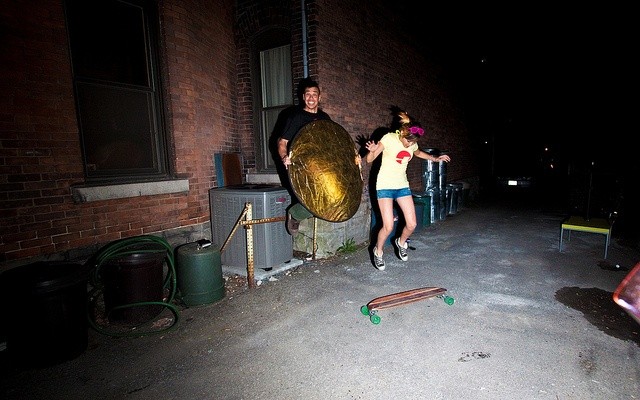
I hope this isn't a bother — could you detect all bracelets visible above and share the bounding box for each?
[281,154,289,161]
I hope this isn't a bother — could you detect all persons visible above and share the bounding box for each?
[365,113,451,271]
[277,82,330,235]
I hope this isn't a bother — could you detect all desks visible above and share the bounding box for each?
[559,215,612,259]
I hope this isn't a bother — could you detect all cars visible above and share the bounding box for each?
[496,151,555,193]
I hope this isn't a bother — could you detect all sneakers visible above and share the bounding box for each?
[288,211,300,237]
[373,246,385,271]
[395,237,408,261]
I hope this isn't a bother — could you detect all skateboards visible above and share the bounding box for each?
[360,286,455,324]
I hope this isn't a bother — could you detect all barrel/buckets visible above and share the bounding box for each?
[411,202,424,233]
[423,192,440,223]
[420,147,439,192]
[0,259,91,371]
[98,240,166,326]
[176,241,224,307]
[439,161,448,190]
[370,207,401,248]
[414,193,431,227]
